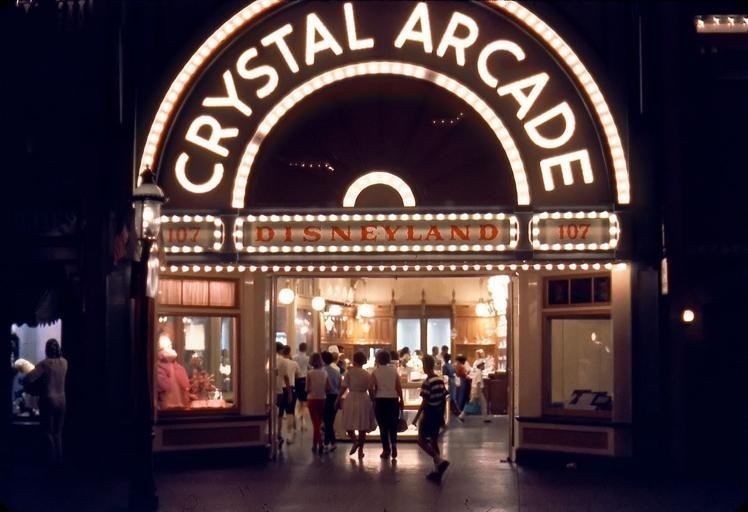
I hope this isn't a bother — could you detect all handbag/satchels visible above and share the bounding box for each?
[464,398,481,415]
[398,409,407,432]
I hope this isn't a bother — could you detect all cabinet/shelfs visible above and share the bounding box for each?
[347,379,425,442]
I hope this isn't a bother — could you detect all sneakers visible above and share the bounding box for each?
[426,461,449,480]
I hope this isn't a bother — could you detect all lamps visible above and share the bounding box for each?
[475,275,508,345]
[279,275,377,331]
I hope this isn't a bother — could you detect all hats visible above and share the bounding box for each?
[328,345,339,353]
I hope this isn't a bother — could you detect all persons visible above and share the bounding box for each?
[266,341,491,483]
[18,339,68,457]
